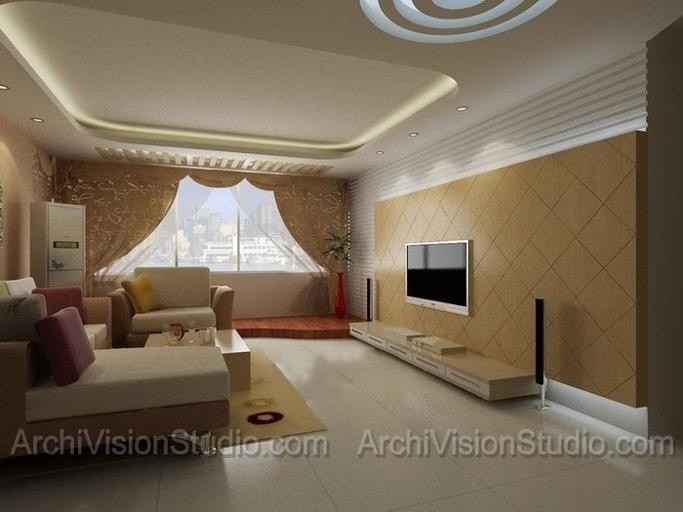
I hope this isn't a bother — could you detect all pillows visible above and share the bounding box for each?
[121,273,164,313]
[32,286,87,325]
[1,292,47,343]
[34,306,96,386]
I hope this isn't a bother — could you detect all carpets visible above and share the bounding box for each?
[0,345,328,479]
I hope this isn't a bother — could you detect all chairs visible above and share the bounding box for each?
[108,267,235,346]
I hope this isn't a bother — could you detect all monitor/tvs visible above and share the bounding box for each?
[404,239,473,317]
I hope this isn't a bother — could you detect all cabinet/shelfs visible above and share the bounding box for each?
[349,321,541,401]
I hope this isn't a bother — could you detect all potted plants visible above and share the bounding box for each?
[322,231,354,319]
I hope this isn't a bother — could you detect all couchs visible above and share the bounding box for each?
[1,277,113,349]
[0,340,230,458]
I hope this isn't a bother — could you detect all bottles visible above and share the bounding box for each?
[184,327,215,346]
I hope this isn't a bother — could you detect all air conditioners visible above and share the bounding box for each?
[30,201,86,298]
